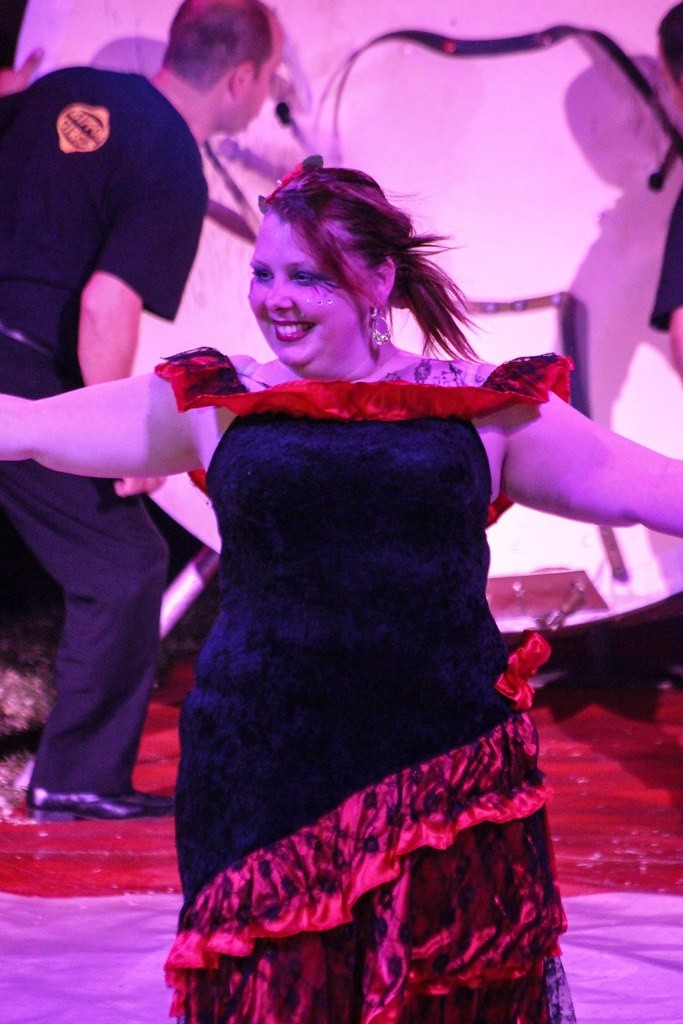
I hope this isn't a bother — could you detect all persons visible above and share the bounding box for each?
[0,151,683,1024]
[0,0,283,818]
[648,1,683,387]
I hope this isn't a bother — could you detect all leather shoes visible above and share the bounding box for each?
[28,786,175,823]
